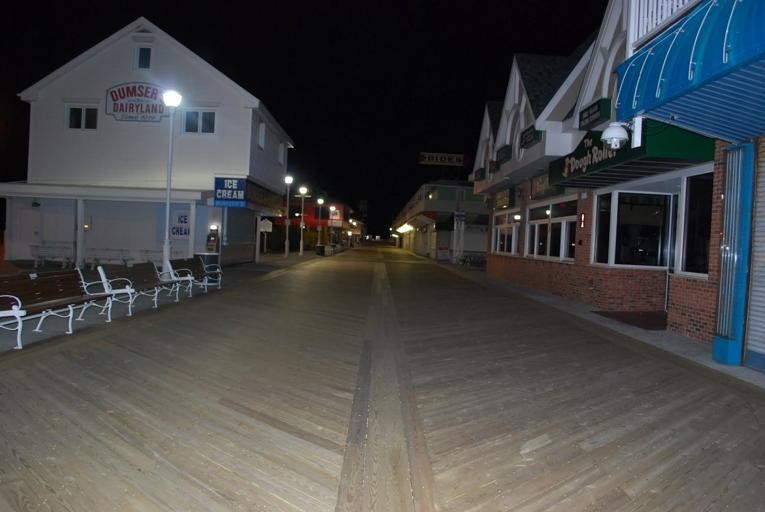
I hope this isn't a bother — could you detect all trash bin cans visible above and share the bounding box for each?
[193,253,219,287]
[315,245,326,256]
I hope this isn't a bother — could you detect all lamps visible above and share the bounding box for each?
[601,121,635,151]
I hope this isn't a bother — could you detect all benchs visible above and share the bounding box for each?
[28,244,74,269]
[0,267,114,349]
[97,261,182,316]
[140,249,185,264]
[85,247,134,270]
[167,257,224,299]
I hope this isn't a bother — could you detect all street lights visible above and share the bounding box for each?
[297,185,307,255]
[159,89,183,284]
[329,206,335,245]
[314,198,325,247]
[283,177,294,258]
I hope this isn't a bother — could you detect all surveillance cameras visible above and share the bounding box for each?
[600,126,629,150]
